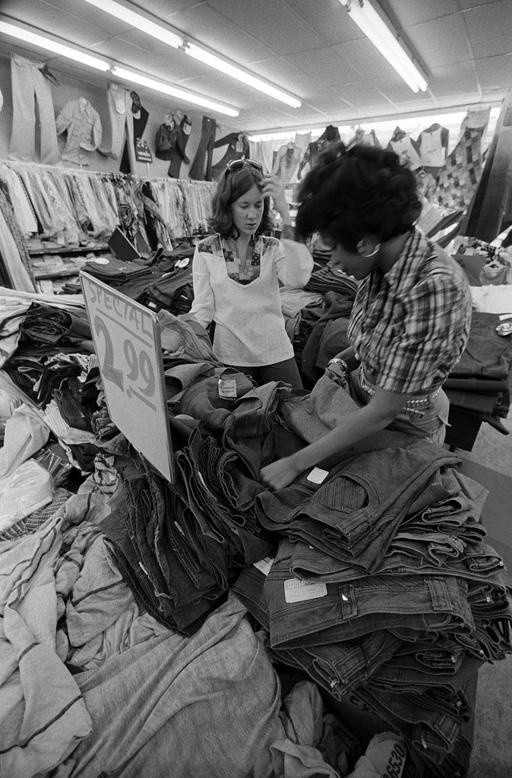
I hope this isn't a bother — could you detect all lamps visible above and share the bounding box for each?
[0,15,110,71]
[86,1,185,55]
[347,0,430,94]
[113,61,241,119]
[185,31,304,110]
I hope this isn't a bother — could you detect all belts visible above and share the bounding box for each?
[358,363,442,420]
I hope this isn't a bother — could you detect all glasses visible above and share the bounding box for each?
[224,158,262,179]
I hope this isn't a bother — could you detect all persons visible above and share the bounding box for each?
[260,143,473,491]
[187,159,313,395]
